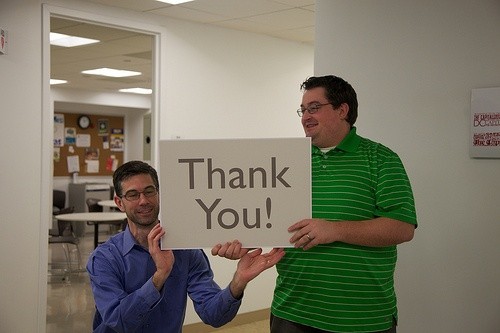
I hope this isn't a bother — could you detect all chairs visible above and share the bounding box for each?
[86,198,121,244]
[49,190,81,282]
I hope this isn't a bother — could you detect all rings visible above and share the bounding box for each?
[306,234,311,240]
[267,260,270,264]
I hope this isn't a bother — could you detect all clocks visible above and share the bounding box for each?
[77,115,91,129]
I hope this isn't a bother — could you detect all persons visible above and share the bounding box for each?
[211,75,419,333]
[85,161,286,333]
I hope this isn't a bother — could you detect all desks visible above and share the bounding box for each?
[55,212,128,250]
[98,200,119,208]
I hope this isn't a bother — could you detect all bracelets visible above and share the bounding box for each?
[152,276,156,284]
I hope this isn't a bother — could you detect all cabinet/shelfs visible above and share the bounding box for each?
[69,183,115,235]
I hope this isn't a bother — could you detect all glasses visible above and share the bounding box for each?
[297,103,332,117]
[120,187,157,200]
